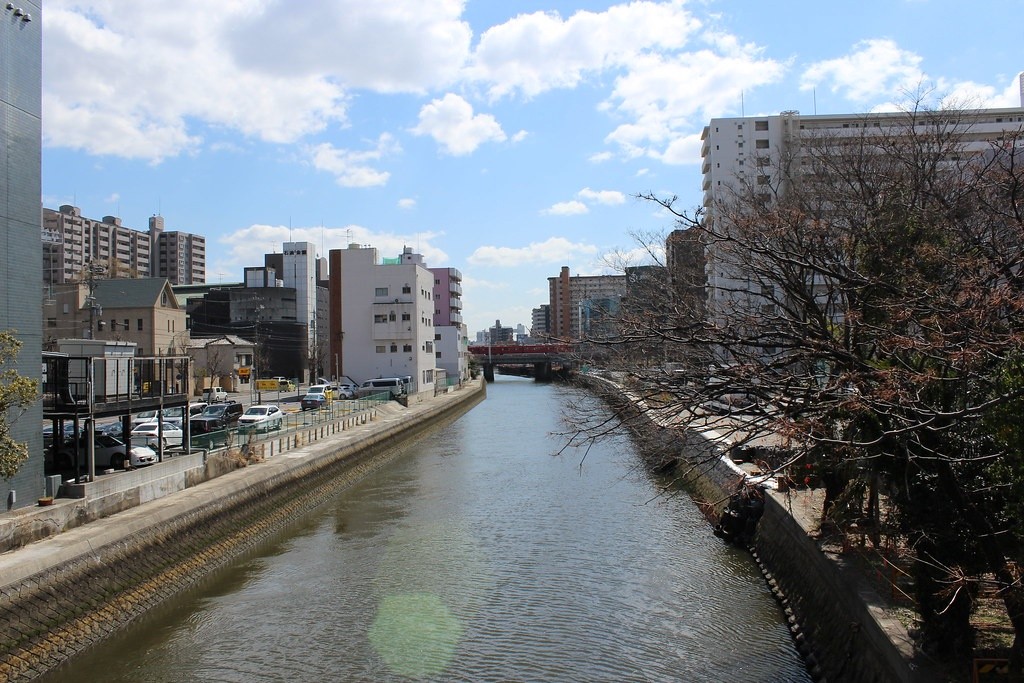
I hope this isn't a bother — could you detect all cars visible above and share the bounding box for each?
[280,380,296,393]
[51,435,156,466]
[307,377,357,400]
[95,421,137,436]
[131,421,183,451]
[300,394,328,411]
[43,423,84,448]
[237,404,283,433]
[133,409,166,427]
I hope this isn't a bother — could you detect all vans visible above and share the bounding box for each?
[358,377,402,397]
[202,387,228,401]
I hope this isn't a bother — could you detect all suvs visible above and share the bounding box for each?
[191,403,243,435]
[164,402,208,429]
